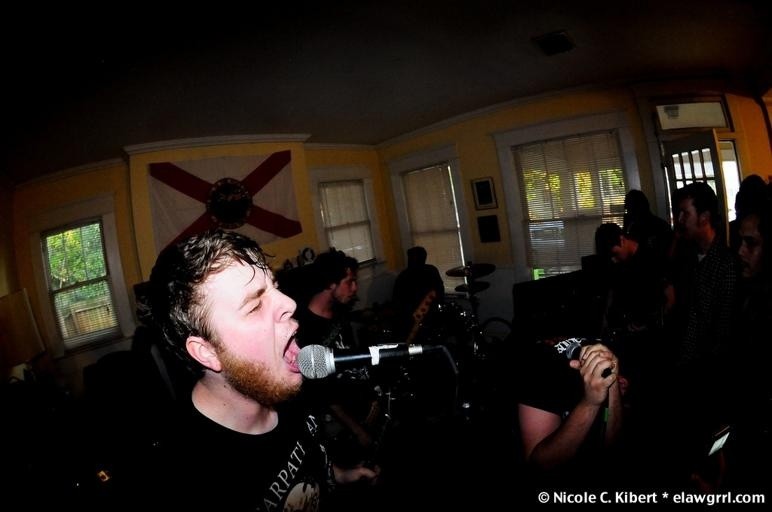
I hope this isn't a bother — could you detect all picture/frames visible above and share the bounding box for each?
[469,176,499,212]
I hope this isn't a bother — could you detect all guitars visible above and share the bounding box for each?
[332,289,443,471]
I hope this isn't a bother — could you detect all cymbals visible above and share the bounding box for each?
[456,281,490,293]
[445,262,496,277]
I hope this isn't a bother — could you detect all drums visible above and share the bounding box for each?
[436,302,466,338]
[422,303,452,343]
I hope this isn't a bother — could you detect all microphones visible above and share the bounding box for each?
[295,343,424,379]
[565,343,613,381]
